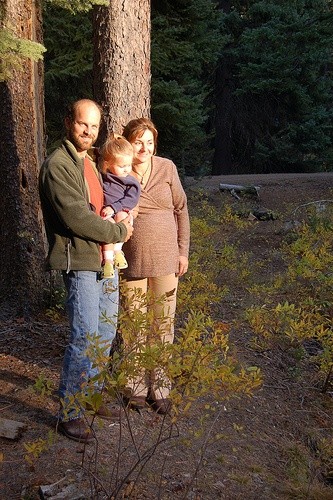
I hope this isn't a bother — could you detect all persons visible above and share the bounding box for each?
[38,98,126,441]
[118,117,190,416]
[94,130,142,281]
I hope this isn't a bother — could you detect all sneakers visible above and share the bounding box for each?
[155,398,173,413]
[86,400,123,420]
[127,395,146,408]
[59,417,94,444]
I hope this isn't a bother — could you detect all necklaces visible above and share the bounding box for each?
[131,159,160,185]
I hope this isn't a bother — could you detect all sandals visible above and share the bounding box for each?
[100,250,128,279]
